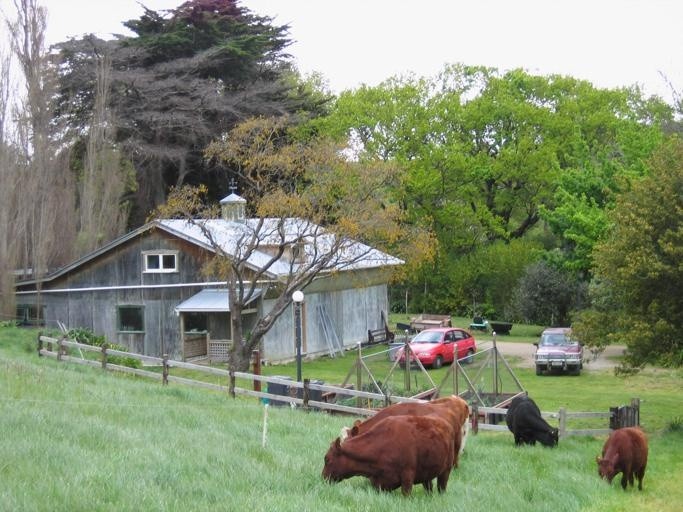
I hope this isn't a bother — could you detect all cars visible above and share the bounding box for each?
[394,326,476,369]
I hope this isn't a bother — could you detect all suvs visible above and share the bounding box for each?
[531,326,585,378]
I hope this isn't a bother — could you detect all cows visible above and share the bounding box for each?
[338,393,471,470]
[320,414,456,500]
[596,426,648,492]
[505,393,559,449]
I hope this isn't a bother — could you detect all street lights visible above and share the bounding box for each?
[291,290,305,400]
